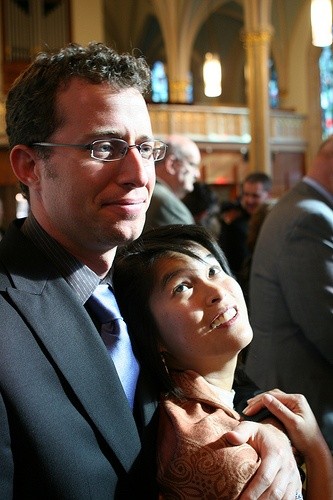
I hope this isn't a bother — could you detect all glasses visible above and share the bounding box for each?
[31,138,168,162]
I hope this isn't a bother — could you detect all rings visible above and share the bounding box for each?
[295,492,303,500]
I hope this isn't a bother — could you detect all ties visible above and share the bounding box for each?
[90,285,140,417]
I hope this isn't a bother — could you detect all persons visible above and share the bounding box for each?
[145,130,333,451]
[0,40,303,500]
[113,224,333,500]
